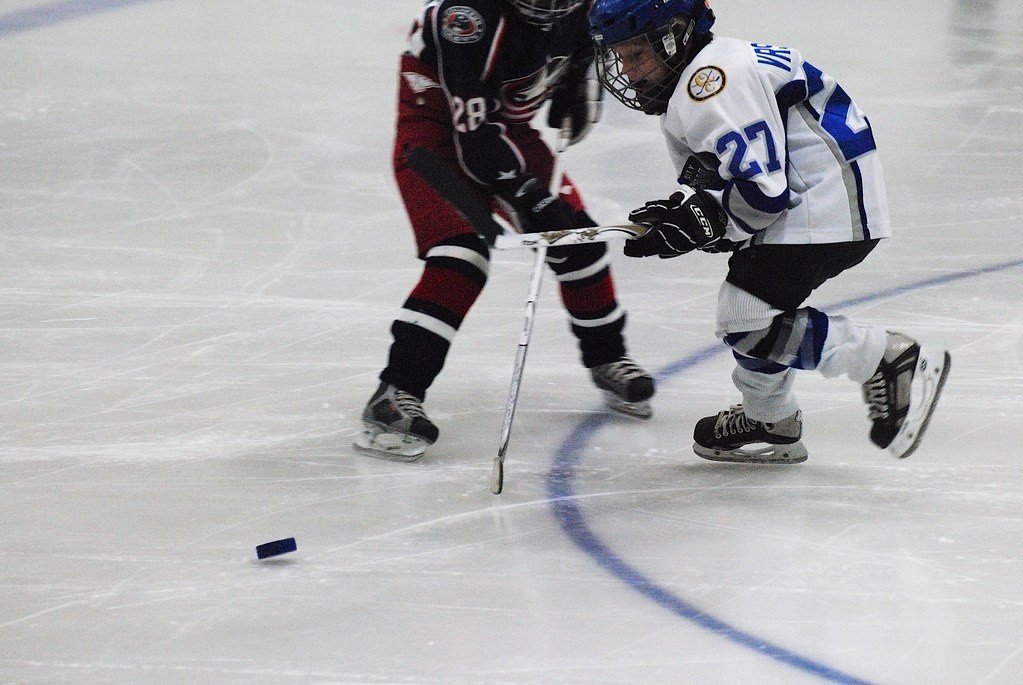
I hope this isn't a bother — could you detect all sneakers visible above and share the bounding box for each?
[353,378,438,463]
[594,357,655,419]
[862,331,950,457]
[692,404,809,465]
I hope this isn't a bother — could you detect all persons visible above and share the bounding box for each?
[587,0,952,464]
[351,0,654,460]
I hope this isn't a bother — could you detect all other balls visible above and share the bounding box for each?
[255,537,298,561]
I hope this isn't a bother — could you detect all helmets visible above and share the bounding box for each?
[587,0,715,116]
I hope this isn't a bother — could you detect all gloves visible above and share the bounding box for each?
[623,191,727,260]
[497,180,585,272]
[548,66,588,145]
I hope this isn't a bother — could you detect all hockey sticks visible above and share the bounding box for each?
[409,144,659,252]
[489,114,572,496]
